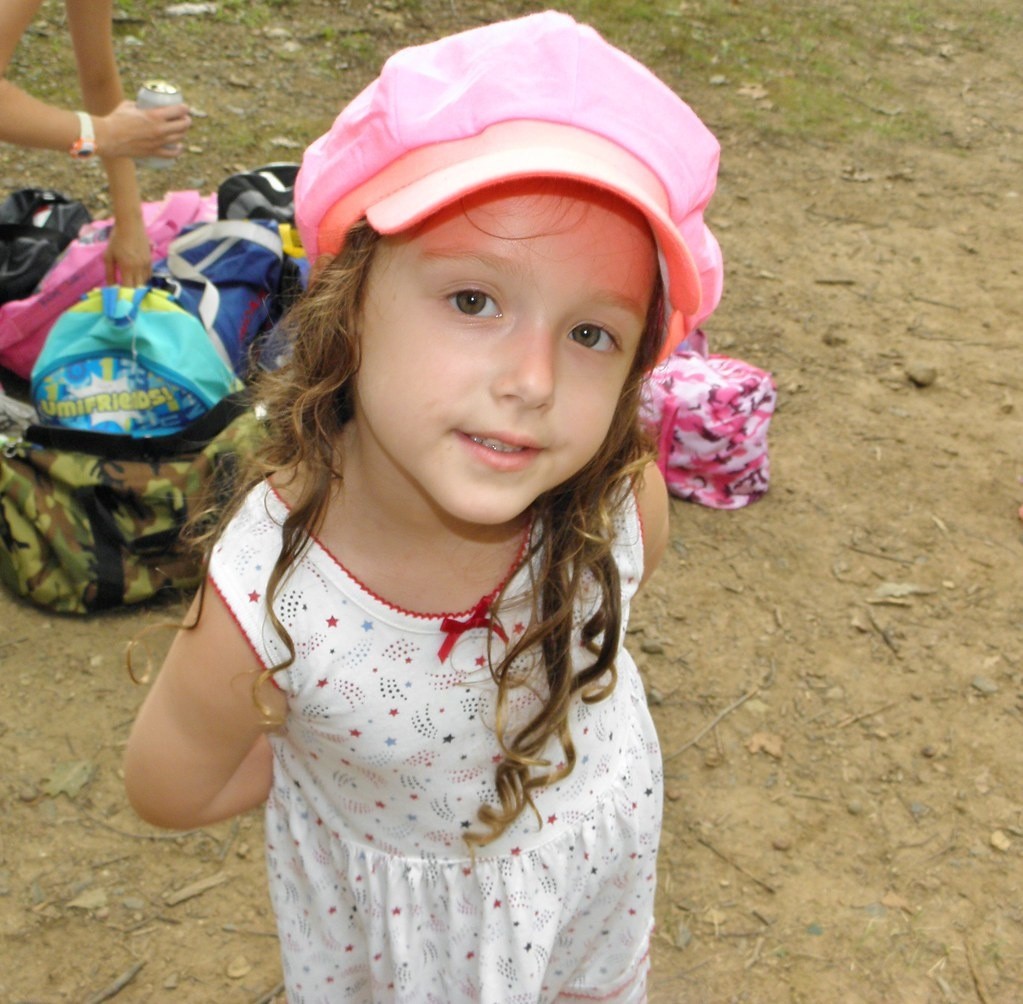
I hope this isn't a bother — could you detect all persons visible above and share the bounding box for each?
[0,0,191,290]
[124,8,721,1004]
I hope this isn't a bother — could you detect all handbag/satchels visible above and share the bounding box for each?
[637,331,776,511]
[0,163,303,614]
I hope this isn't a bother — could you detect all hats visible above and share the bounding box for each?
[294,9,723,375]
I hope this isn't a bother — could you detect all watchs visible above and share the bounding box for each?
[70,112,97,158]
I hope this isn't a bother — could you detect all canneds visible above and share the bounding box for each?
[133,79,184,169]
